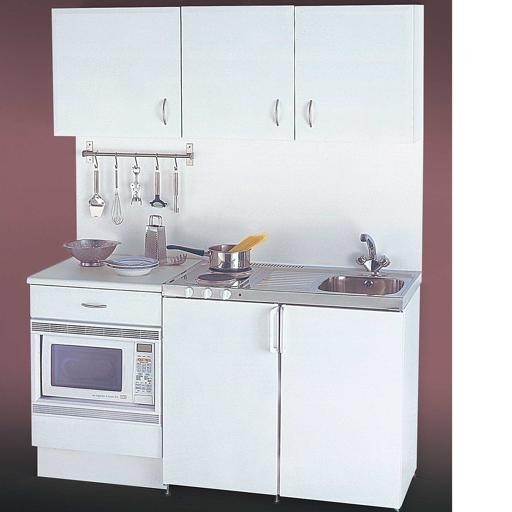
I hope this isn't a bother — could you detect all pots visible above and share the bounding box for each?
[164,243,253,273]
[62,237,124,263]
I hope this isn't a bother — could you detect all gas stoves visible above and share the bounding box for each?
[163,258,269,300]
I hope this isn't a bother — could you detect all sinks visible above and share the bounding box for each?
[318,274,405,297]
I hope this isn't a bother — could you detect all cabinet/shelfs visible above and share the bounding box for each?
[181,5,425,142]
[162,286,421,511]
[51,7,182,135]
[28,285,162,329]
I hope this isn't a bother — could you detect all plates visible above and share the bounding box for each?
[102,257,160,278]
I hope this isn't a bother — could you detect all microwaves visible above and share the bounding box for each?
[38,331,157,410]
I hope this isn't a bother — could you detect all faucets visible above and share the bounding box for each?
[355,233,391,274]
